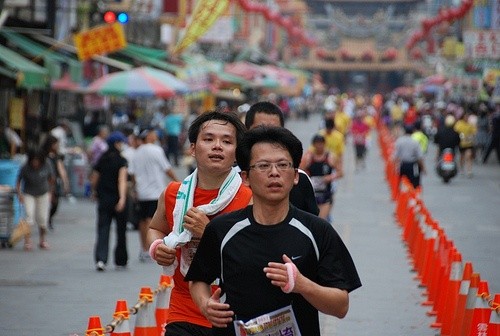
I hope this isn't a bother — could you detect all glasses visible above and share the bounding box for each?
[251,161,294,171]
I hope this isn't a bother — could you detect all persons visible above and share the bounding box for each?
[245,103,320,216]
[299,135,343,219]
[184,126,362,336]
[147,113,253,336]
[317,92,500,188]
[6,114,186,271]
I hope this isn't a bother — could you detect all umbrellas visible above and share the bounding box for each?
[88,63,317,96]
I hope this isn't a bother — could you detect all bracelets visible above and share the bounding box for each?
[149,239,164,260]
[281,262,296,294]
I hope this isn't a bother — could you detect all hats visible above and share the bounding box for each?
[105,131,129,145]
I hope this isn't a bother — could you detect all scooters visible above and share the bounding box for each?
[437,146,459,181]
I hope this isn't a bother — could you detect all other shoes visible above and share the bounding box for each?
[38,242,49,248]
[139,251,152,262]
[23,243,32,250]
[116,265,128,271]
[97,261,105,271]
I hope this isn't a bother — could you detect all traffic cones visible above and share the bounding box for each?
[131,287,161,336]
[108,300,131,336]
[467,281,492,335]
[155,274,172,331]
[378,119,462,335]
[450,262,474,335]
[487,293,500,336]
[86,316,106,336]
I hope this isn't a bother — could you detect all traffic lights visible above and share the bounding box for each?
[91,8,129,25]
